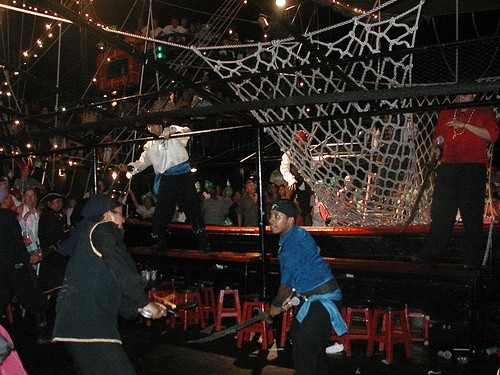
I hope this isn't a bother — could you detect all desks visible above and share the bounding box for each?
[269,254,482,335]
[130,247,273,298]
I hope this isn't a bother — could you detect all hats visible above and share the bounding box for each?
[40,194,66,203]
[144,114,165,125]
[271,199,298,217]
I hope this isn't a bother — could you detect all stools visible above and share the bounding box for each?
[143,273,430,364]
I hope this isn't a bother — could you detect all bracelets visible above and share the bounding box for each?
[272,298,282,307]
[463,123,466,128]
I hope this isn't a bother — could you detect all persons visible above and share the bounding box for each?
[315,175,367,226]
[0,175,76,326]
[264,182,303,225]
[426,92,500,265]
[52,195,167,375]
[118,117,212,253]
[281,130,313,226]
[197,180,259,227]
[268,199,349,375]
[84,179,107,198]
[130,190,155,218]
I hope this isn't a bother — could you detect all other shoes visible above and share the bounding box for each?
[199,245,213,253]
[150,244,168,252]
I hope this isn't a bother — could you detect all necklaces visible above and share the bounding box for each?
[453,109,475,140]
